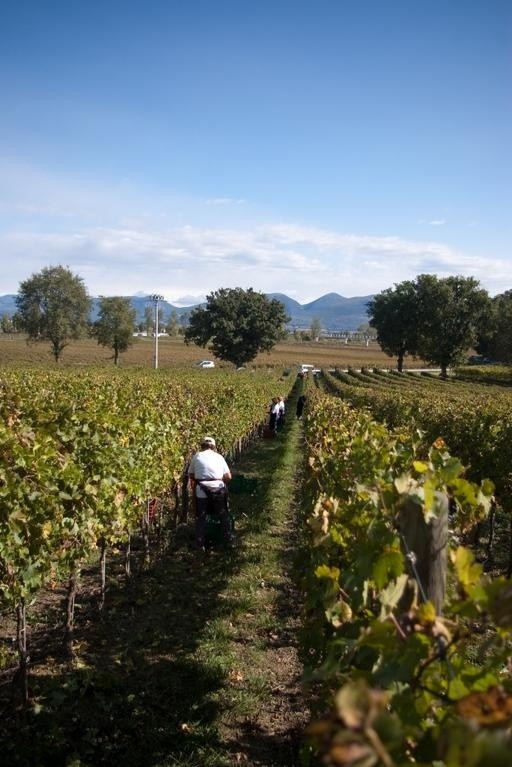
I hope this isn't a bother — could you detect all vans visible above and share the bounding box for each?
[297,364,315,373]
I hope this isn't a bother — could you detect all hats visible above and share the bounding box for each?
[201,436,216,447]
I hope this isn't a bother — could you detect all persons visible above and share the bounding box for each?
[188,436,233,549]
[277,396,286,428]
[270,396,279,434]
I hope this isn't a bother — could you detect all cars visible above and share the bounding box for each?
[192,360,215,368]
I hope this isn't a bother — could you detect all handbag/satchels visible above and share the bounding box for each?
[200,483,228,501]
